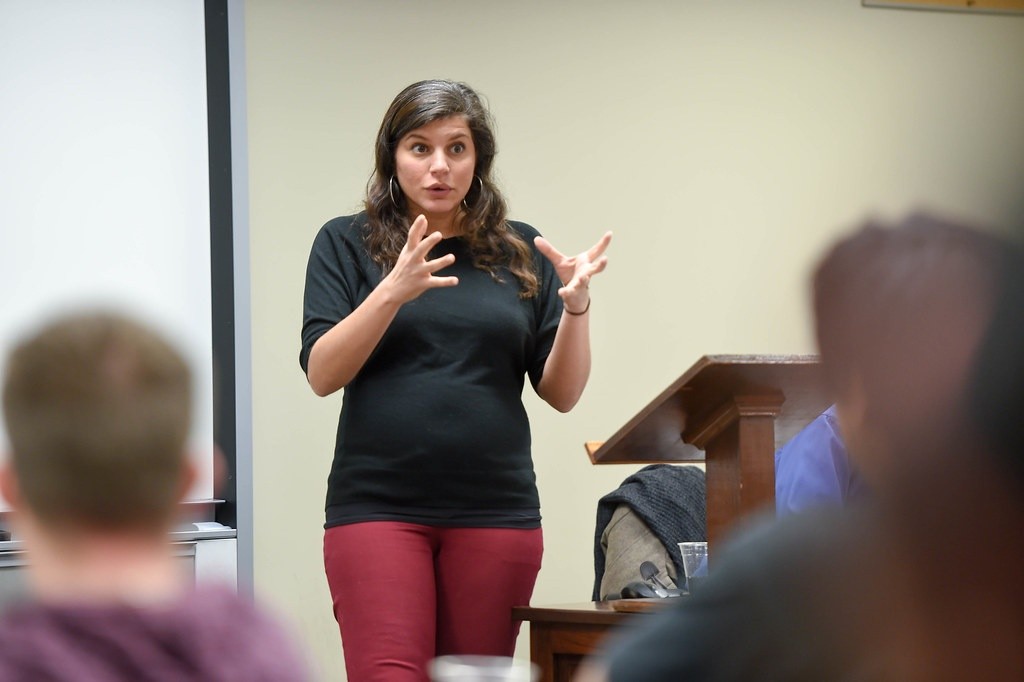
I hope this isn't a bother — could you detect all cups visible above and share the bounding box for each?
[678,542,708,593]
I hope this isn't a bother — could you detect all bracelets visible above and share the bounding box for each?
[563,297,590,316]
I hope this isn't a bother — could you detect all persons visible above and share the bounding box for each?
[0,304,314,682]
[569,205,1024,682]
[299,81,613,682]
